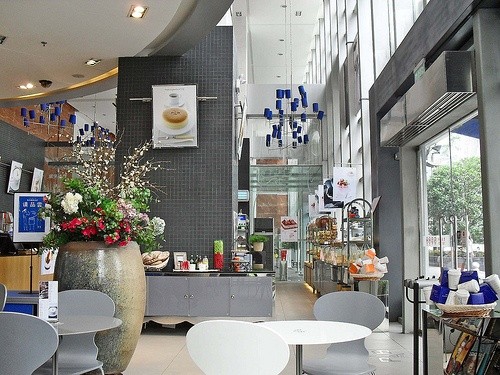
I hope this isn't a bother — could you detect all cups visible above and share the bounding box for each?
[422,267,500,305]
[199,264,205,270]
[190,264,196,270]
[168,92,180,105]
[348,248,389,274]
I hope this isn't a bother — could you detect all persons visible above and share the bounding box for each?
[460,231,473,273]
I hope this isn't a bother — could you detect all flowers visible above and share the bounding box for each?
[37,122,171,259]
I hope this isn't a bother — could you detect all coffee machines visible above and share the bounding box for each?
[2,211,13,237]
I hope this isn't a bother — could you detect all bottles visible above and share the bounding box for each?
[177,261,190,270]
[189,255,194,264]
[203,256,208,270]
[199,256,203,263]
[194,255,199,270]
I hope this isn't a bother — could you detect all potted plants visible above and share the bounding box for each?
[248,236,269,251]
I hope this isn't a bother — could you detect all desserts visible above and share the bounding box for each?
[162,109,189,128]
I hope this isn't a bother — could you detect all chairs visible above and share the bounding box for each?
[303,291,386,375]
[0,312,60,375]
[186,320,290,375]
[33,290,116,375]
[0,283,6,311]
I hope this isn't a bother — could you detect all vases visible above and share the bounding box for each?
[53,239,146,375]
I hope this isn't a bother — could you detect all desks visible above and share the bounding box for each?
[256,320,372,375]
[47,317,123,375]
[423,308,500,375]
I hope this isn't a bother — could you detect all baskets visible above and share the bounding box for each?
[351,274,384,280]
[435,300,498,318]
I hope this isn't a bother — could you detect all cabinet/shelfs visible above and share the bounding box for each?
[304,199,373,295]
[142,271,273,325]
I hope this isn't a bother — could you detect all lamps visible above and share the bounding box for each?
[264,0,324,149]
[21,80,111,149]
[234,74,245,94]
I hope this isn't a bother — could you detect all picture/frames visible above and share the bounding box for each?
[31,167,44,192]
[7,160,23,195]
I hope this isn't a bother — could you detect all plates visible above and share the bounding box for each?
[162,100,184,107]
[155,107,194,136]
